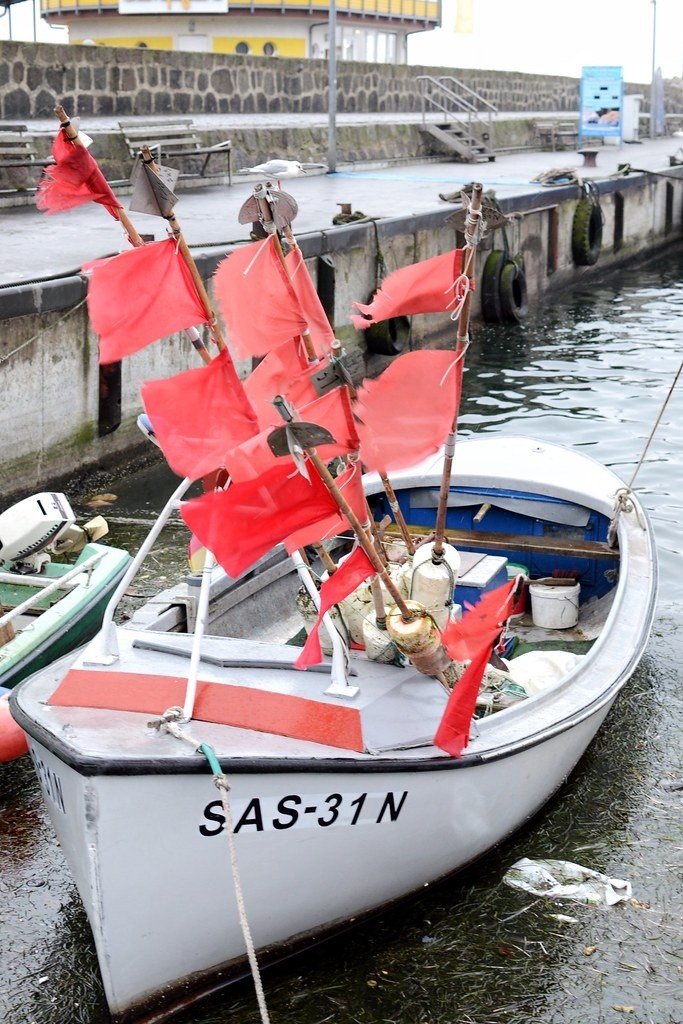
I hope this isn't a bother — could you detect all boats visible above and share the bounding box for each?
[0,493,136,755]
[5,101,659,1017]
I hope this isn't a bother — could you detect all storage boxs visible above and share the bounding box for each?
[453,551,509,613]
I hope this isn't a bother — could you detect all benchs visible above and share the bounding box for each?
[536,120,578,151]
[1,124,57,191]
[348,523,619,573]
[120,119,233,191]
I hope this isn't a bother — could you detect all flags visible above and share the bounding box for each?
[213,237,307,359]
[442,576,528,663]
[140,349,260,480]
[238,338,321,440]
[347,248,464,330]
[220,386,360,485]
[281,466,368,555]
[78,237,213,363]
[282,248,337,359]
[351,350,461,474]
[34,129,122,219]
[294,546,376,668]
[176,451,340,583]
[434,635,496,761]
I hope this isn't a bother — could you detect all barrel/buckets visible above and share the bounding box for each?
[529,577,579,630]
[506,562,529,619]
